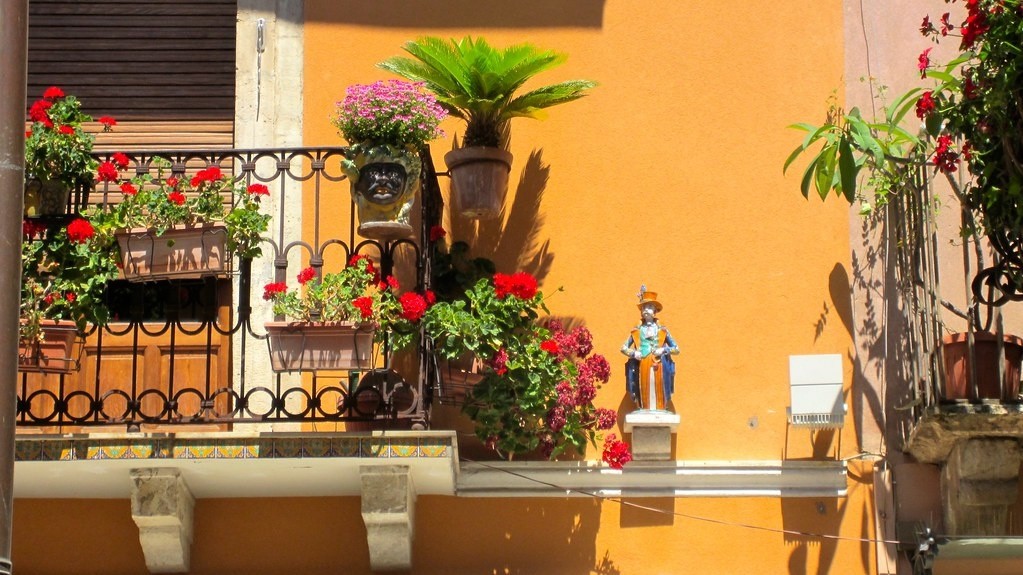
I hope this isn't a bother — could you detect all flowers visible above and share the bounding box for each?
[424,227,633,471]
[328,79,449,147]
[20,156,273,360]
[25,87,130,189]
[915,0,1023,262]
[262,253,435,356]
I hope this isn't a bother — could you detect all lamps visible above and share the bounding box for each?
[784,354,848,462]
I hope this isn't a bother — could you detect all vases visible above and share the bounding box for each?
[18,318,78,375]
[341,141,422,240]
[438,335,487,397]
[263,321,373,370]
[115,222,228,281]
[24,178,71,219]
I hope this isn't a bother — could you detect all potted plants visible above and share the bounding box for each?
[783,54,1023,404]
[375,36,599,219]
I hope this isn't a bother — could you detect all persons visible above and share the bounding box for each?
[621,291,680,413]
